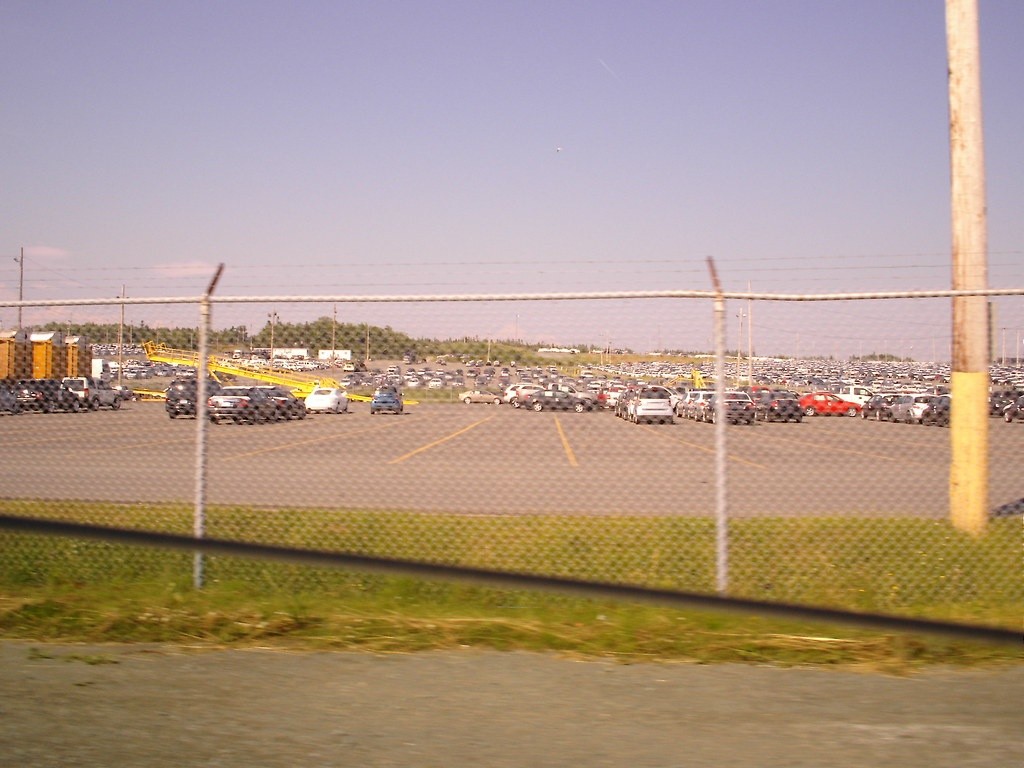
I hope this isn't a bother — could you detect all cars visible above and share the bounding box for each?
[0,379,25,414]
[457,389,505,405]
[206,386,279,425]
[258,386,307,421]
[89,359,199,386]
[372,384,398,399]
[371,391,405,416]
[303,387,349,414]
[111,384,132,401]
[524,388,593,415]
[218,347,1024,426]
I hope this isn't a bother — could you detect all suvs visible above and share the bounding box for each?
[58,377,122,411]
[8,377,94,414]
[164,379,219,419]
